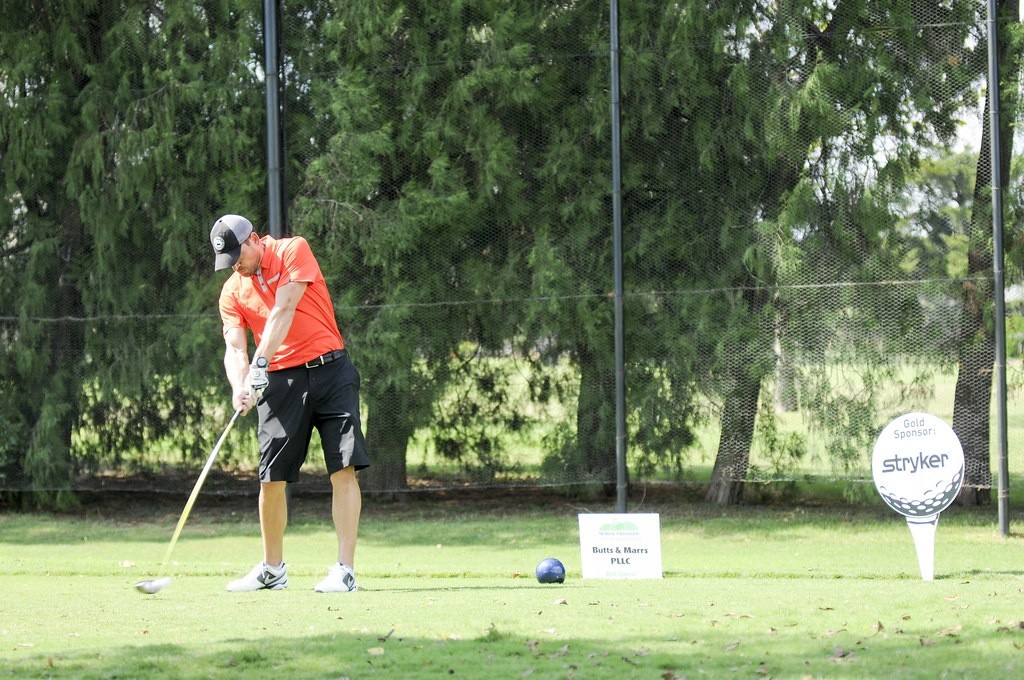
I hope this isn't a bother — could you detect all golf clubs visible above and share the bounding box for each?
[134,371,268,594]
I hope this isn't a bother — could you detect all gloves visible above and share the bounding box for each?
[245,367,270,398]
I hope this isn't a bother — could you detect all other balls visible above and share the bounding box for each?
[535,557,566,584]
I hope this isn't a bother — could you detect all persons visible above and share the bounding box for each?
[210,214,369,592]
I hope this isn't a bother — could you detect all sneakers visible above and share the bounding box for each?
[313,562,358,592]
[226,561,290,592]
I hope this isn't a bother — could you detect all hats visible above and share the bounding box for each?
[209,214,254,273]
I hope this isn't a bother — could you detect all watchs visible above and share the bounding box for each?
[252,357,269,368]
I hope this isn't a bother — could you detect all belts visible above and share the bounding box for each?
[293,348,349,369]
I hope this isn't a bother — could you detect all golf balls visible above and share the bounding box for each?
[152,576,172,589]
